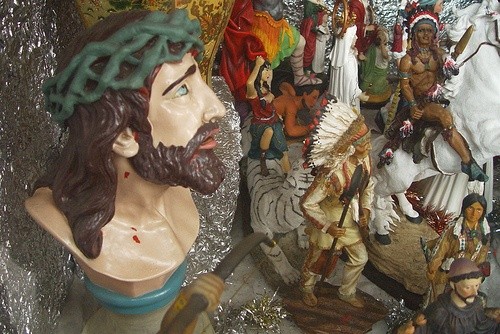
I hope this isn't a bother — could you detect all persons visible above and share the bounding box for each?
[219,0,392,137]
[413,258,500,334]
[160,272,225,334]
[426,193,491,305]
[399,0,490,182]
[246,56,292,177]
[25,9,226,299]
[299,93,374,308]
